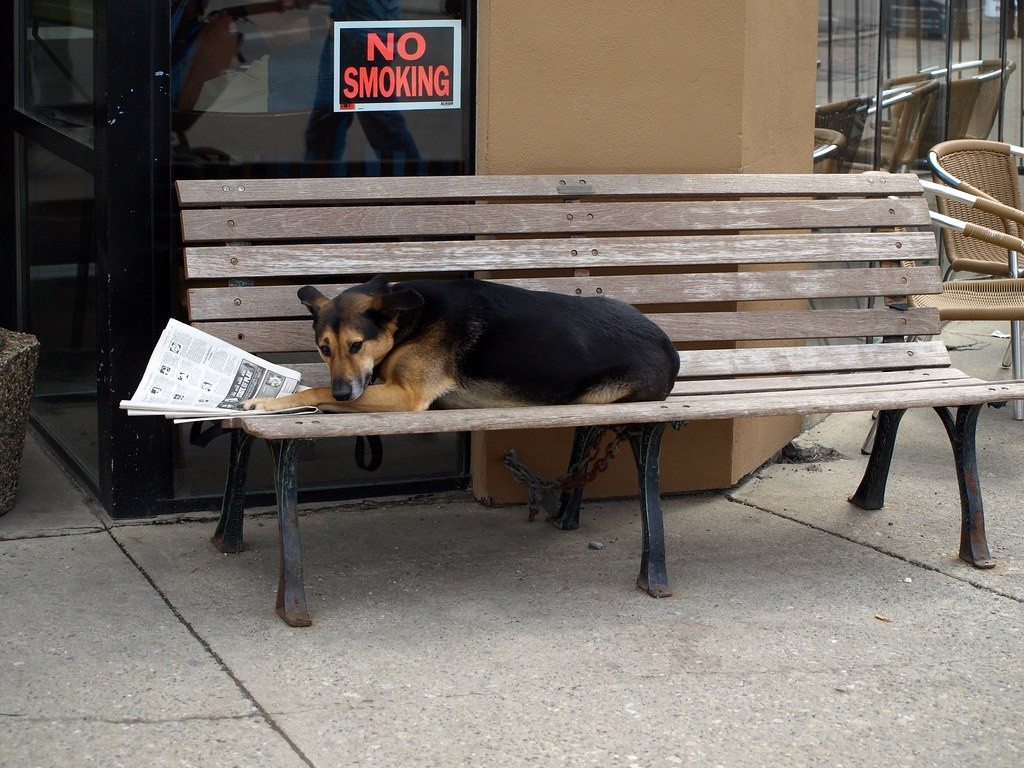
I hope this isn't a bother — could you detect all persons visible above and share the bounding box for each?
[304,0,423,176]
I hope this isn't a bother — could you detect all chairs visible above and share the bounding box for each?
[928,139,1024,367]
[860,169,1024,455]
[812,57,1016,200]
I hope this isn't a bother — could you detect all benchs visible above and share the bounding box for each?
[176,172,1024,627]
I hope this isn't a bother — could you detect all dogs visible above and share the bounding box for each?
[236,273,681,411]
